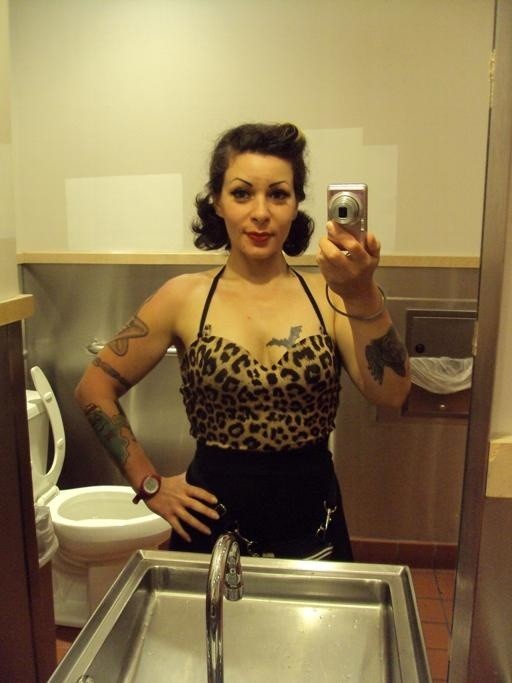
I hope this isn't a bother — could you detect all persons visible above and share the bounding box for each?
[75,118,410,568]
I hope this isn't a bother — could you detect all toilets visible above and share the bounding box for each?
[25,365,172,630]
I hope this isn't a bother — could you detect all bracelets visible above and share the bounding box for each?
[324,281,390,320]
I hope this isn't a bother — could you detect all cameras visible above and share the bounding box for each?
[328,183,367,250]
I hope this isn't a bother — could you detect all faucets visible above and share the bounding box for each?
[205,532,244,682]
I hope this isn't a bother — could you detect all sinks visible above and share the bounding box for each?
[46,548,434,682]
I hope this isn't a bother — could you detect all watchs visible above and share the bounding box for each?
[129,471,160,504]
